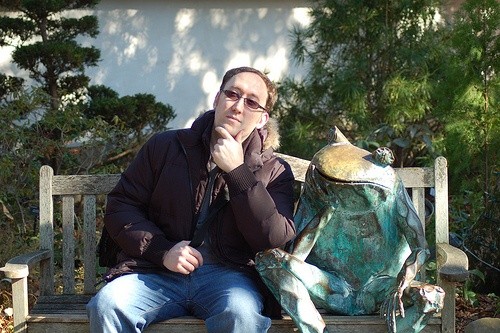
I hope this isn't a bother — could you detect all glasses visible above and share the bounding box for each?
[223,90,266,111]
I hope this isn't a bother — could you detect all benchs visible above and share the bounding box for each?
[4,151,469,333]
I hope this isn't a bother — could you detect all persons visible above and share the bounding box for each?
[85,67,298,333]
